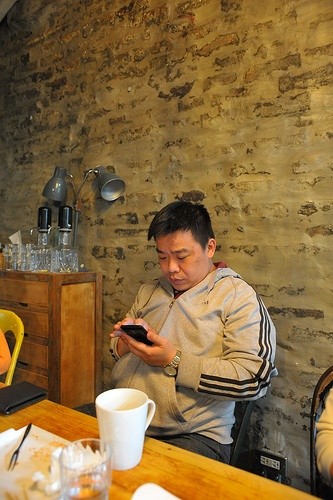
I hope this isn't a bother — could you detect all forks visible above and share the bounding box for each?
[7,422,32,471]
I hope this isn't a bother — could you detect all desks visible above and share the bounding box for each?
[0,381,323,500]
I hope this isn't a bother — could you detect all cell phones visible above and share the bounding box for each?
[120,325,153,347]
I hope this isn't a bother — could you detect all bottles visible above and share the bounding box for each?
[28,230,79,272]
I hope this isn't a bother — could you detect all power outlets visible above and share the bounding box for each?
[254,448,288,478]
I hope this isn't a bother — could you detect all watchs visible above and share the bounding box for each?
[163,350,181,377]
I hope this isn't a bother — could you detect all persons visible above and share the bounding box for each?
[110,201,278,464]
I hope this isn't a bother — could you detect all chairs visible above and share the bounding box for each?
[0,310,24,385]
[310,365,333,500]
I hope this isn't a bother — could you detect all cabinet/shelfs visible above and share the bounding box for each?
[0,271,103,409]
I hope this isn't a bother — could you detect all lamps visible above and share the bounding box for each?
[42,164,126,272]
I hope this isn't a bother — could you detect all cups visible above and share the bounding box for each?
[61,438,113,500]
[95,388,156,472]
[4,242,35,271]
